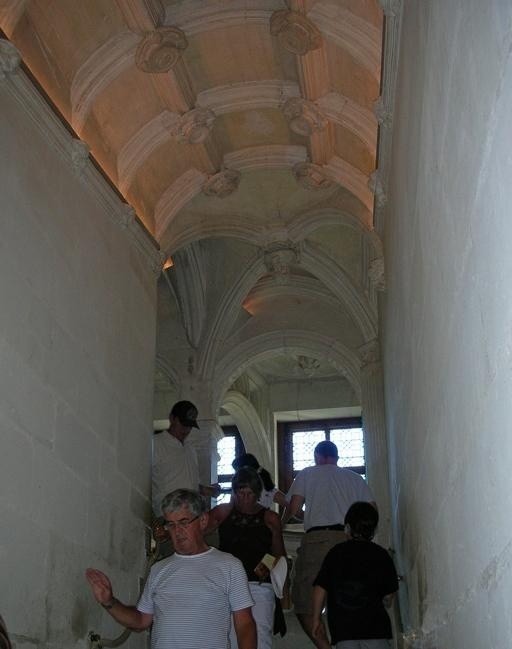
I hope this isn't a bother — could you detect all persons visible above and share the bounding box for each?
[312,501,400,649]
[151,399,222,562]
[152,466,288,649]
[84,486,261,649]
[283,439,376,649]
[230,453,304,522]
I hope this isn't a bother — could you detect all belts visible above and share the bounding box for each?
[306,525,345,533]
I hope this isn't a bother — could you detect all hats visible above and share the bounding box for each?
[173,400,200,430]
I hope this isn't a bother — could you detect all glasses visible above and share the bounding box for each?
[166,515,199,529]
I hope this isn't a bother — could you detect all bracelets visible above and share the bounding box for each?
[101,598,119,610]
[153,524,161,530]
